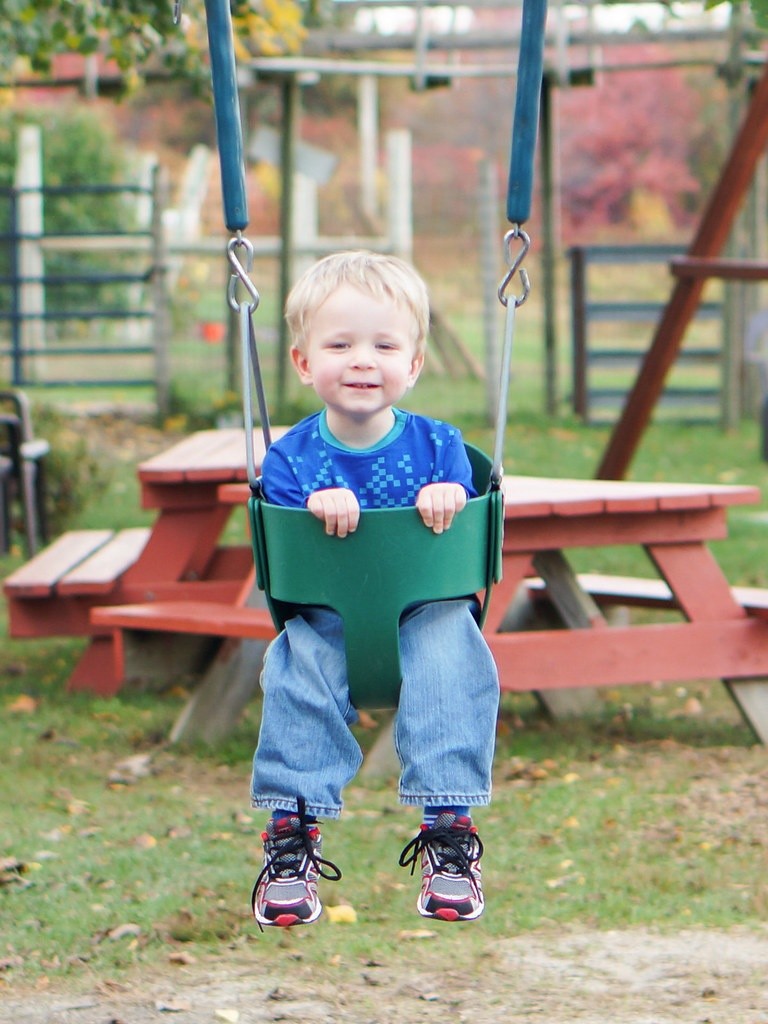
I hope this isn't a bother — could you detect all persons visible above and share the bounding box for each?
[250,250,491,926]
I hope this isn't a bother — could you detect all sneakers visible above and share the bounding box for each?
[399,812,486,921]
[250,795,342,933]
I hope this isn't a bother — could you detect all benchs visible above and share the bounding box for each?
[85,601,279,645]
[532,571,768,624]
[3,524,149,595]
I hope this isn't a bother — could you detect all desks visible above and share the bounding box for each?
[67,425,297,693]
[173,472,767,785]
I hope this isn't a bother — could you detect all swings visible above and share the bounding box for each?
[202,0,548,714]
